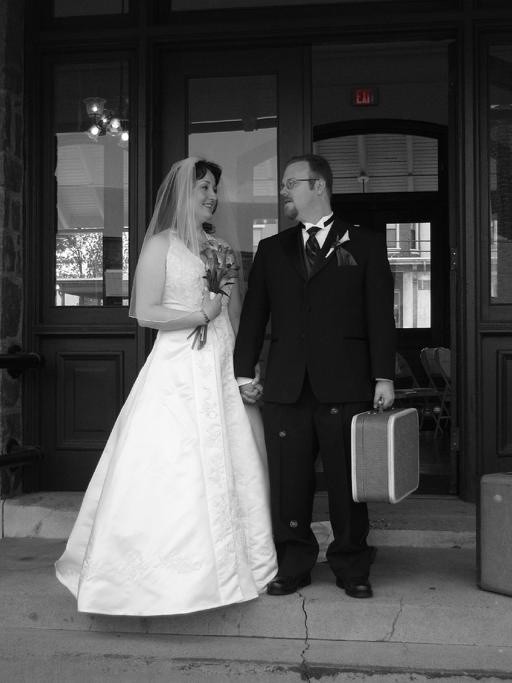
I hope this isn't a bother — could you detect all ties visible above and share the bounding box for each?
[306,226,321,281]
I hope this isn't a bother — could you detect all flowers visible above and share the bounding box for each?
[185,240,241,351]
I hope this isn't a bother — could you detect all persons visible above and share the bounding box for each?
[232,155,397,599]
[54,158,280,618]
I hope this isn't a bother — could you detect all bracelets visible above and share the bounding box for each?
[200,309,211,324]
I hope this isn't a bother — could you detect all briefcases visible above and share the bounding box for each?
[350,399,420,505]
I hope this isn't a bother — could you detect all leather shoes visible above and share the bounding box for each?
[268,571,313,596]
[337,571,373,599]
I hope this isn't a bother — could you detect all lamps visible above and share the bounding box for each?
[80,0,131,150]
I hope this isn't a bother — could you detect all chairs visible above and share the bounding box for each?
[393,346,453,441]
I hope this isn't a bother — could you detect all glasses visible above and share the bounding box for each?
[280,178,319,189]
